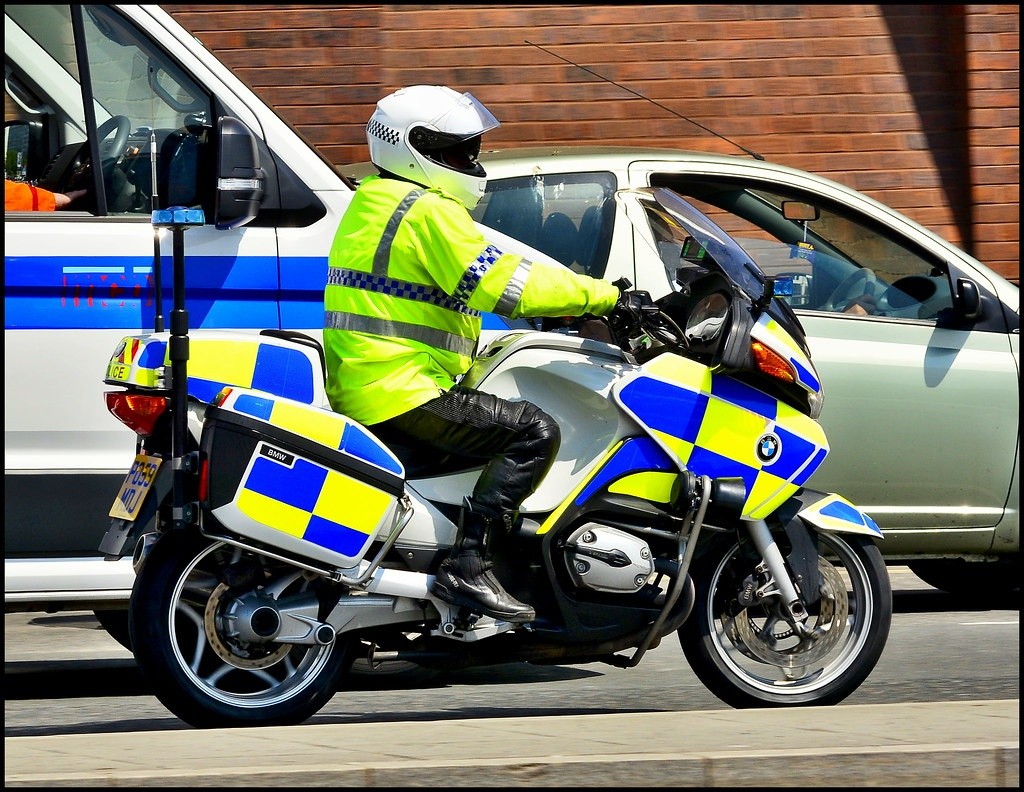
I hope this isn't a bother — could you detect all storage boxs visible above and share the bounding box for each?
[197,386,406,568]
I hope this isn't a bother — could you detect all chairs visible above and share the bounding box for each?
[544,212,578,269]
[576,205,601,273]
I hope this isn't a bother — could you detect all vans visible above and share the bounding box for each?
[5,4,356,654]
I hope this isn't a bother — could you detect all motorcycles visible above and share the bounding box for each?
[98,186,893,729]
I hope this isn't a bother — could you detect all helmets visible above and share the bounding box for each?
[364,84,489,212]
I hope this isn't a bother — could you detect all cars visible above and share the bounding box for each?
[350,40,1020,589]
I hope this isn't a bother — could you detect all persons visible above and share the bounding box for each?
[643,201,875,315]
[5,179,87,211]
[324,84,642,622]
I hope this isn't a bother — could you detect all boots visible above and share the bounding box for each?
[429,495,536,623]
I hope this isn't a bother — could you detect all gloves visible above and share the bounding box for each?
[610,290,643,340]
